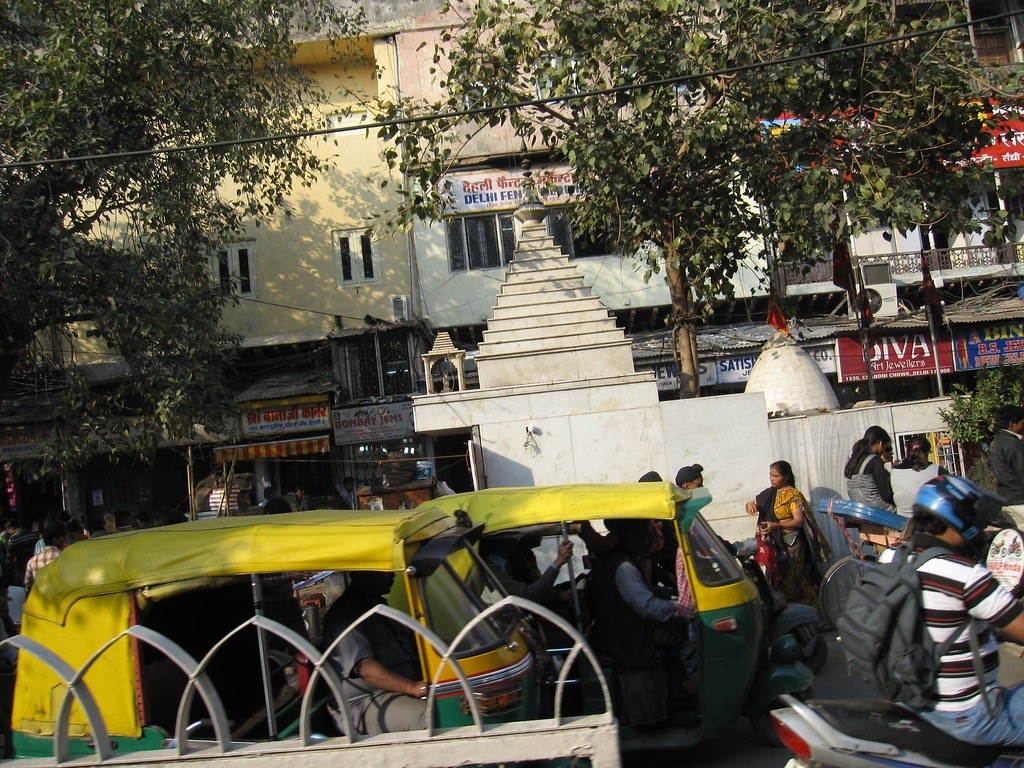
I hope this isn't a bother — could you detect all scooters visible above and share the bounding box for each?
[769,693,1005,768]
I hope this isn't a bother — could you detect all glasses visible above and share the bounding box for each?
[885,447,893,453]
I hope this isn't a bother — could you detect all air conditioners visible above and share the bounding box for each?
[847,283,899,319]
[390,294,408,322]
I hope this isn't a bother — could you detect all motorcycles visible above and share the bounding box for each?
[7,508,550,757]
[414,483,827,752]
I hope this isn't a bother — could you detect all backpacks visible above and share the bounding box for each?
[814,543,972,711]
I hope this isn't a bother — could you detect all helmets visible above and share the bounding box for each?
[913,474,1007,546]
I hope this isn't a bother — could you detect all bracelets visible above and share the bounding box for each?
[732,549,739,559]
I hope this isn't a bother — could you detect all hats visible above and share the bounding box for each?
[676,464,703,487]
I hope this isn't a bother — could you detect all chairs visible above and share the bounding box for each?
[580,571,665,670]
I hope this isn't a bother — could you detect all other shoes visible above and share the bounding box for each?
[674,693,696,706]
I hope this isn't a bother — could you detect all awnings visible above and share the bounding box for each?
[213,435,329,463]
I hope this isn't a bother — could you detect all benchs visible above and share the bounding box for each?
[141,608,302,740]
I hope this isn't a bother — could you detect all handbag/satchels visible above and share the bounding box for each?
[756,531,778,571]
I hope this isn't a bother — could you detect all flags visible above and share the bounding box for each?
[765,281,790,334]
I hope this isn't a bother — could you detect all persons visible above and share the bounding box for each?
[316,476,355,510]
[844,426,901,514]
[262,487,292,515]
[878,474,1024,749]
[296,485,313,511]
[745,461,832,618]
[651,464,747,585]
[483,536,574,609]
[890,436,949,519]
[988,405,1024,506]
[0,511,187,665]
[317,570,427,735]
[576,518,699,692]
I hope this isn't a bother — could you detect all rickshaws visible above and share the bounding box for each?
[819,498,910,633]
